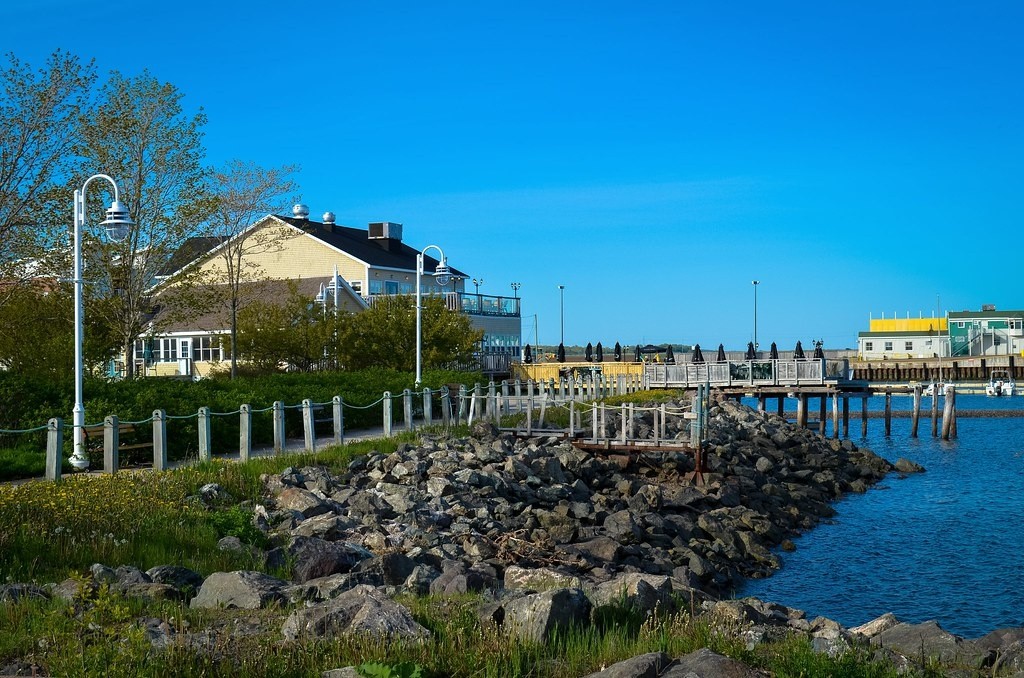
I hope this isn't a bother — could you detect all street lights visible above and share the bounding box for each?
[752,281,759,352]
[413,245,451,389]
[558,285,565,345]
[67,172,136,475]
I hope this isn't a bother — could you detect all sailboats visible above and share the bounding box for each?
[923,294,949,396]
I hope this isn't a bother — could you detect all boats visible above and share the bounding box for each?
[986,368,1015,396]
